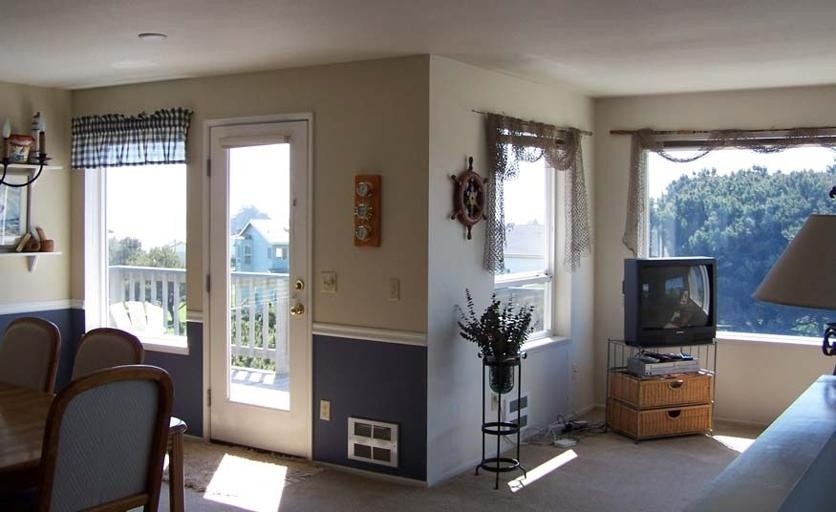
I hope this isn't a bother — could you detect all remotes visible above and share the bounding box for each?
[640,355,660,363]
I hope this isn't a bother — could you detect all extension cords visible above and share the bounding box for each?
[549,420,587,431]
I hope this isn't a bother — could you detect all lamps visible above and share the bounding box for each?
[748,205,836,357]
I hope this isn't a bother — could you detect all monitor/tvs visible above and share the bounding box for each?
[624,256,718,348]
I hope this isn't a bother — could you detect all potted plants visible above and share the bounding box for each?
[454,286,538,391]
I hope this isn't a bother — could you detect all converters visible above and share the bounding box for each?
[554,439,576,448]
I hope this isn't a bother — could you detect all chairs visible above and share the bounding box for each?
[0,299,177,512]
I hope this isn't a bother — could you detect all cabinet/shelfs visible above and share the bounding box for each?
[0,162,66,270]
